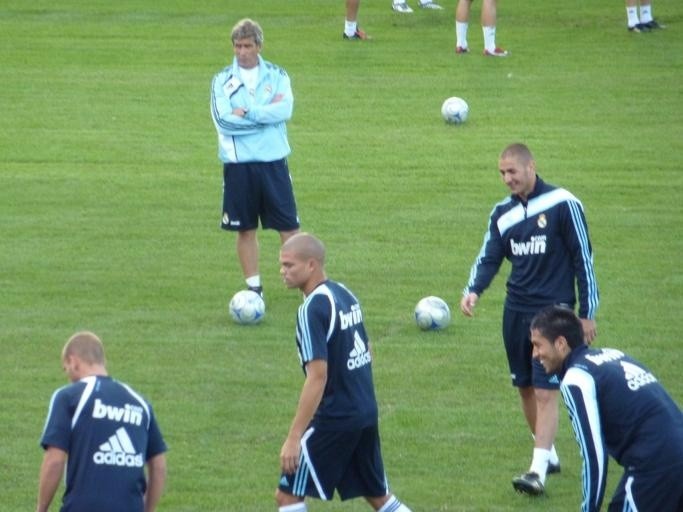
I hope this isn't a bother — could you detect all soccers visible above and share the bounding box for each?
[414,297,449,331]
[441,97,468,122]
[228,290,264,323]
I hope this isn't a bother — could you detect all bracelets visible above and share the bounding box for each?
[242,107,249,118]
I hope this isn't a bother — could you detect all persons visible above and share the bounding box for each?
[391,0,443,15]
[526,308,682,512]
[623,0,667,34]
[35,329,169,512]
[341,1,369,41]
[454,0,509,58]
[206,17,303,299]
[272,232,413,512]
[459,142,597,498]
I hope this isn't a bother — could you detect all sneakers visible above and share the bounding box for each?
[645,21,668,31]
[343,31,372,41]
[547,460,560,473]
[511,471,549,500]
[482,47,508,57]
[417,1,444,11]
[627,23,656,34]
[248,284,264,300]
[455,43,471,54]
[391,2,413,14]
